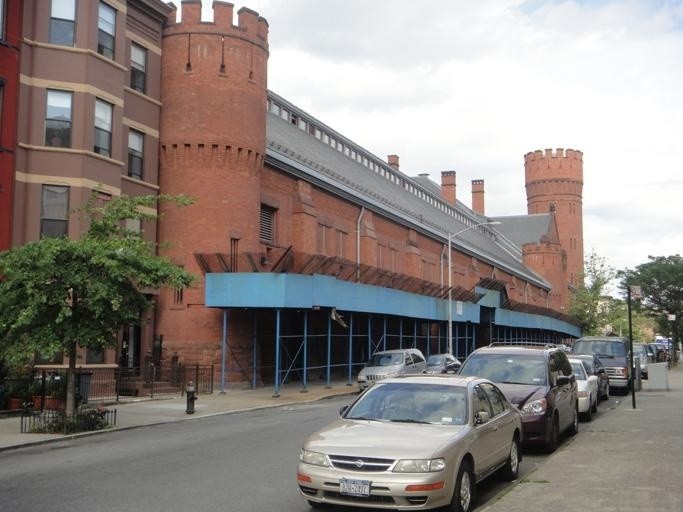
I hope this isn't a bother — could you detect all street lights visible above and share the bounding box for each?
[447,220,504,358]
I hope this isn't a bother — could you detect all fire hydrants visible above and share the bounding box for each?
[182,379,199,415]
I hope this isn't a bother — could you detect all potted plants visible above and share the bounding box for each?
[8,370,82,414]
